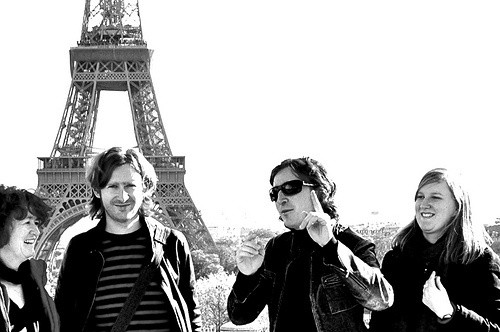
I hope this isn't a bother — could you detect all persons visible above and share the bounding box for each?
[53,147,202,332]
[0,185,61,332]
[368,168,500,332]
[226,157,393,332]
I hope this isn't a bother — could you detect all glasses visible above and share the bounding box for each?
[269,180,316,202]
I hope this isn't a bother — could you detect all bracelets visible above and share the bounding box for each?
[442,314,452,319]
